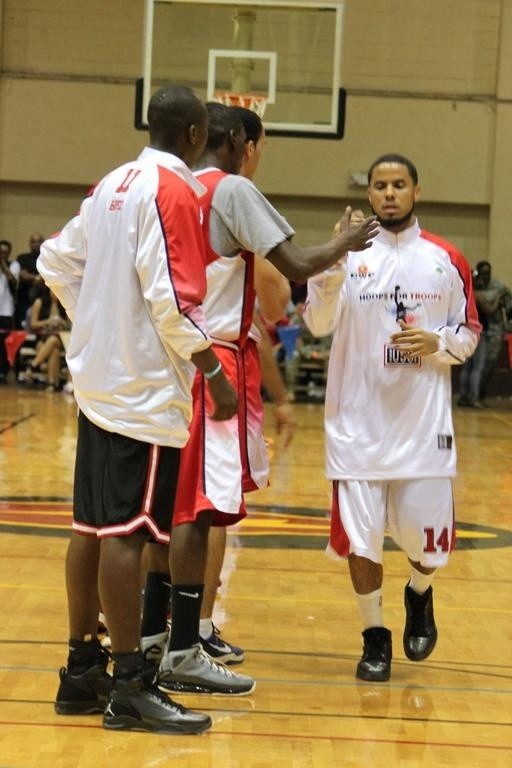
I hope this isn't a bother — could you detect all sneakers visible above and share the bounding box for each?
[54,620,255,733]
[457,395,469,406]
[403,579,437,660]
[356,626,392,681]
[468,397,487,408]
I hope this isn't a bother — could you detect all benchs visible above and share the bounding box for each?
[17,333,65,390]
[280,360,326,400]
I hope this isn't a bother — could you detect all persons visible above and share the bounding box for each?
[33,83,380,735]
[458,260,511,410]
[0,232,74,396]
[302,154,482,684]
